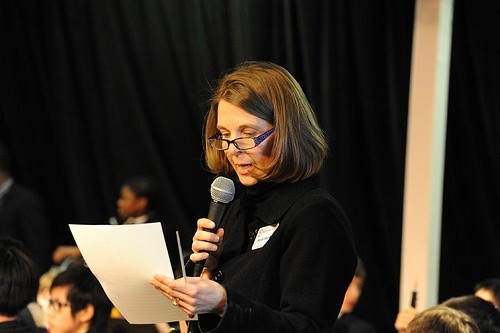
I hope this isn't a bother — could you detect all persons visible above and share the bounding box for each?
[151,60,358,333]
[0,148,500,333]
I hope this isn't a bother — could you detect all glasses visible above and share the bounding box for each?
[206,127,276,152]
[47,299,71,311]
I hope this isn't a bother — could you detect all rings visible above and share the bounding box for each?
[171,298,180,305]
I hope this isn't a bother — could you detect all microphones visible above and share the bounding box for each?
[191,176,236,276]
